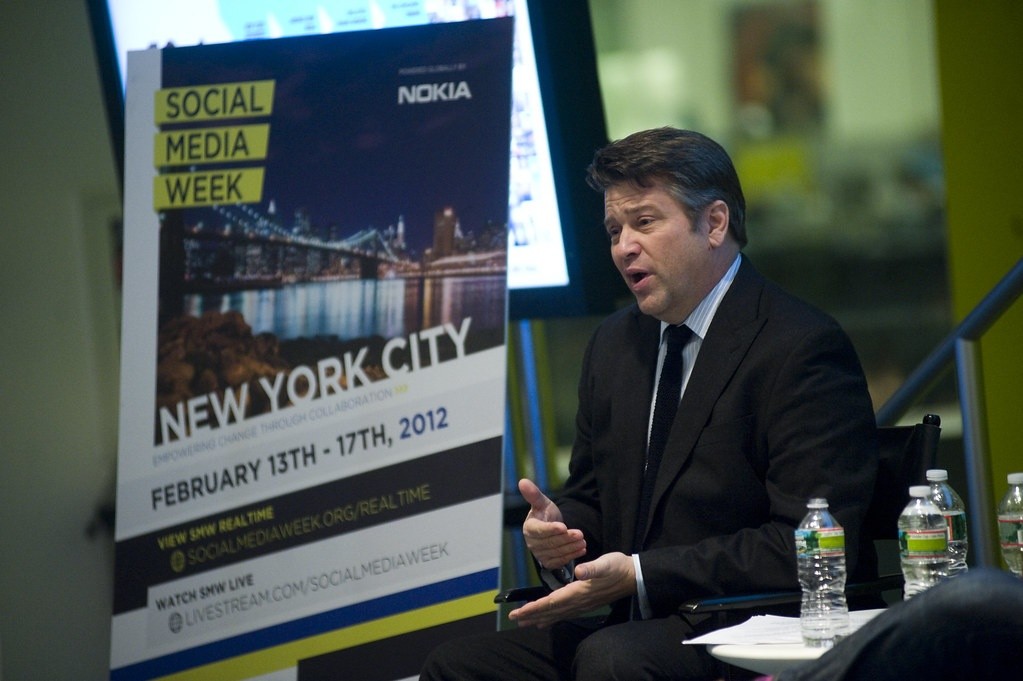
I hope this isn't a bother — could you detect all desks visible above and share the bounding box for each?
[706,609,889,676]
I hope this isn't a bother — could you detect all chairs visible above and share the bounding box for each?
[494,415,940,681]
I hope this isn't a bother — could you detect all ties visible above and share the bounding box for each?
[633,324,694,554]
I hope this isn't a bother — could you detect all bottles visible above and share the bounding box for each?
[996,471,1023,578]
[795,497,851,646]
[924,469,969,578]
[897,485,949,604]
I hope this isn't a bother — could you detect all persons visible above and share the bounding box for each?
[418,125,884,681]
